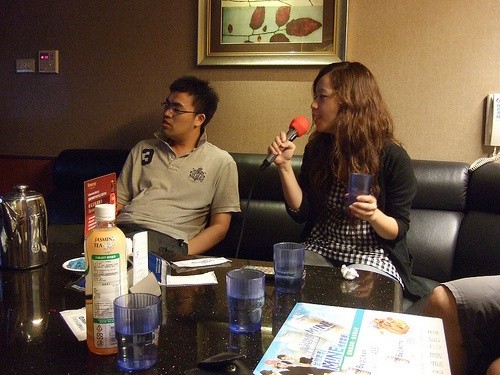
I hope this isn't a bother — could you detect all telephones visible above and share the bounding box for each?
[484,92,500,147]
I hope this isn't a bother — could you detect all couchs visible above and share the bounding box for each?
[44,149,500,311]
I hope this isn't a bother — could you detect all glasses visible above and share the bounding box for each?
[163,102,204,114]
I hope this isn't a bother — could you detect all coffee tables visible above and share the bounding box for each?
[0,241,402,375]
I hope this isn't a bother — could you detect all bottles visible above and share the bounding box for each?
[83,204,127,355]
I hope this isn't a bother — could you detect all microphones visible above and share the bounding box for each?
[259,116,310,171]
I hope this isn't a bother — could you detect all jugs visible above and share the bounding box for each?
[0,185,47,269]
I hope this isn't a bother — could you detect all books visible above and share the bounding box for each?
[148,252,171,325]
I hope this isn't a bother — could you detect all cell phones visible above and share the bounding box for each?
[69,277,85,294]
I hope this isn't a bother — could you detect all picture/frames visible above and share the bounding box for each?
[197,0,349,66]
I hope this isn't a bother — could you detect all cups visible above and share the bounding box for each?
[114,293,162,370]
[347,173,371,205]
[226,269,265,333]
[273,242,306,294]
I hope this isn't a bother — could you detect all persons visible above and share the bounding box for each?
[268,62,430,298]
[403,273,500,375]
[163,269,216,324]
[116,75,241,256]
[273,264,401,315]
[260,354,334,375]
